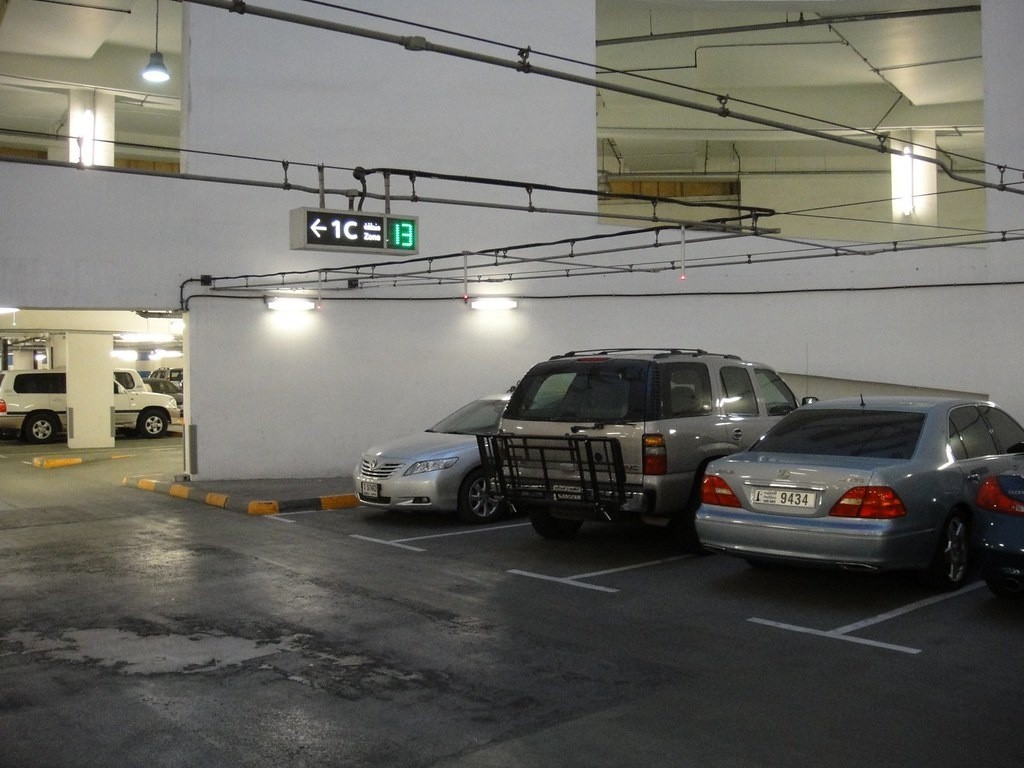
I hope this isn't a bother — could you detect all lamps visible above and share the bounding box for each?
[142,0,169,82]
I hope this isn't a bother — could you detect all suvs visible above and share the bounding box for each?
[148,365,184,405]
[495,347,820,540]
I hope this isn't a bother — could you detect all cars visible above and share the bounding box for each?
[962,459,1023,594]
[692,396,1024,592]
[0,369,181,445]
[143,378,184,419]
[353,390,512,526]
[113,368,147,393]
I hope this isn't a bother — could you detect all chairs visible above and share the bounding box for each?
[669,386,697,414]
